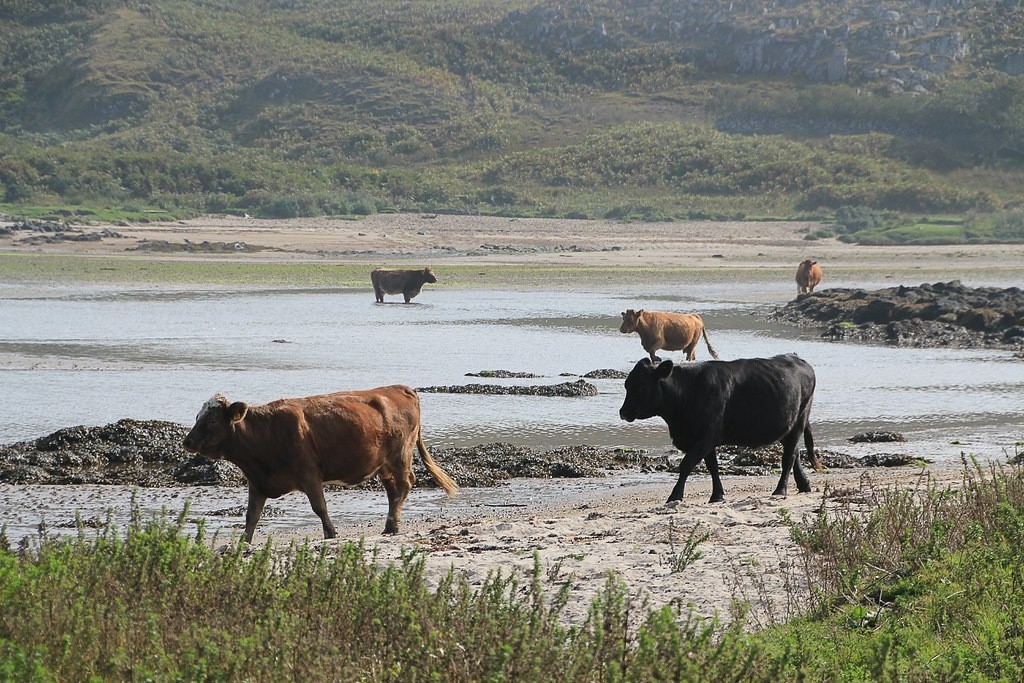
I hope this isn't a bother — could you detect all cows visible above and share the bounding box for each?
[371,267,437,303]
[183,384,460,554]
[620,353,822,505]
[795,259,822,294]
[620,309,718,362]
